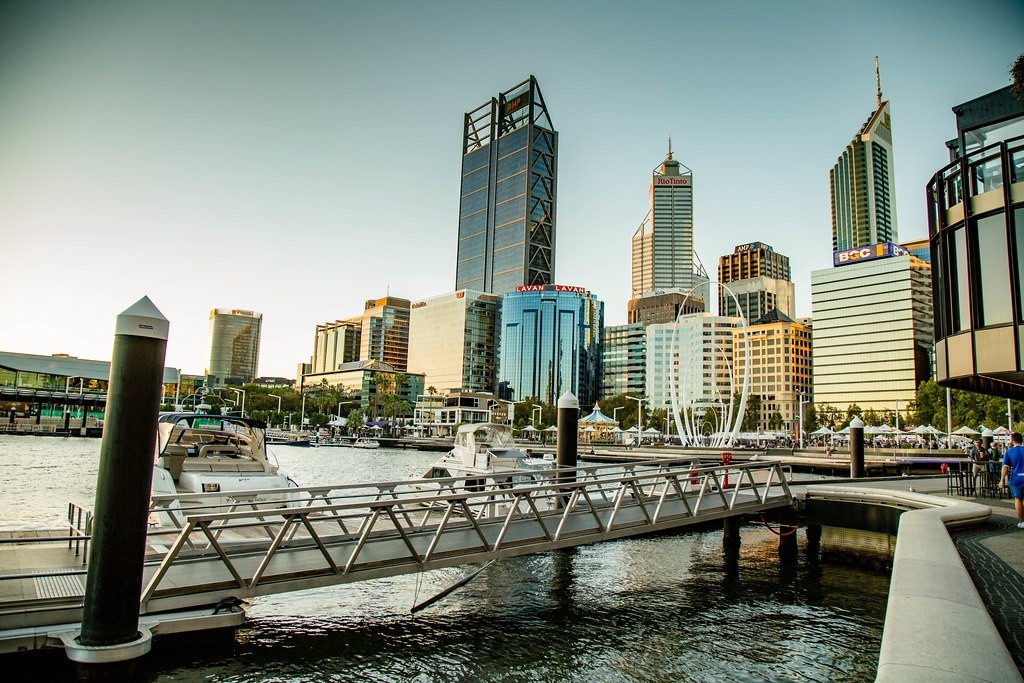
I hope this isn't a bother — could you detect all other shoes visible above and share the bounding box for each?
[1017,521,1024,528]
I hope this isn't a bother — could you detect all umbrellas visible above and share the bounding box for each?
[522,424,1015,454]
[326,420,427,437]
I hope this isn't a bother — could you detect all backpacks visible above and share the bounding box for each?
[991,446,999,462]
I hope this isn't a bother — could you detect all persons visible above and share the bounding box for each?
[968,439,1001,493]
[749,434,1024,457]
[998,432,1024,528]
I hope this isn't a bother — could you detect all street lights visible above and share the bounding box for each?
[79,377,84,396]
[613,407,625,423]
[63,376,80,420]
[532,404,542,430]
[224,386,246,419]
[624,395,650,447]
[795,388,806,449]
[337,401,354,433]
[532,408,543,428]
[499,399,527,438]
[269,394,281,430]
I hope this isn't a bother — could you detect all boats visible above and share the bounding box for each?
[396,422,555,517]
[152,411,310,523]
